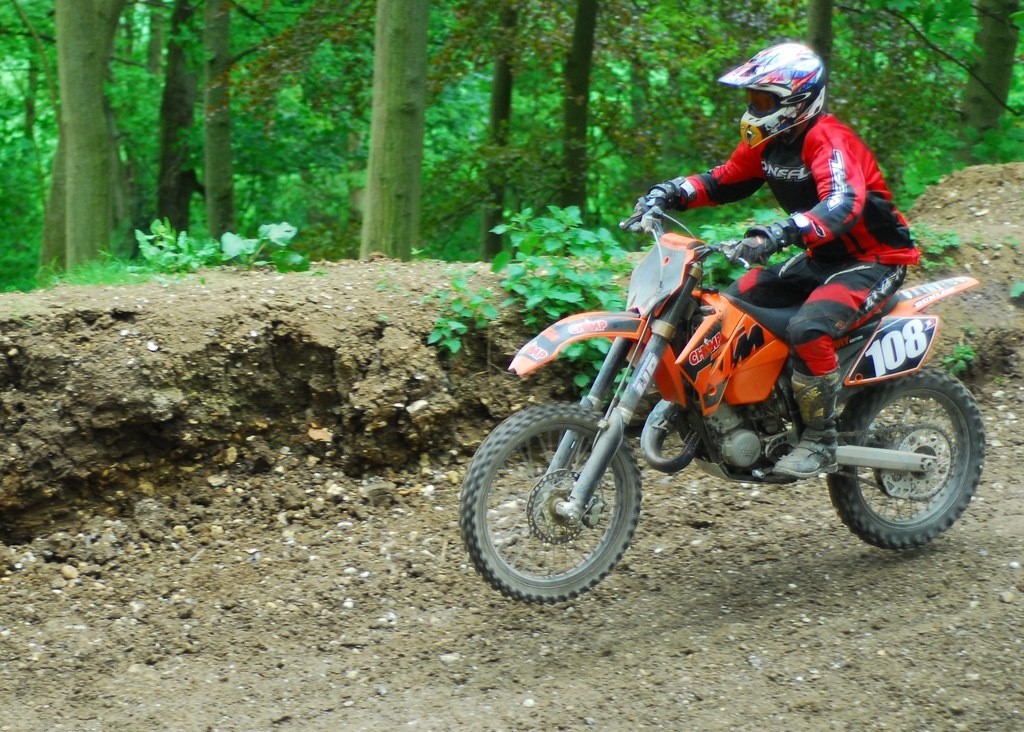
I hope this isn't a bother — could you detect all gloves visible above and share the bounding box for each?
[633,179,678,229]
[730,223,789,267]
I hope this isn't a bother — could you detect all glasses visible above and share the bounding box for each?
[745,88,779,117]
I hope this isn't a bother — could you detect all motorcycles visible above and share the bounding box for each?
[456,189,992,608]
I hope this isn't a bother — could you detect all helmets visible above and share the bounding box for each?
[717,43,825,145]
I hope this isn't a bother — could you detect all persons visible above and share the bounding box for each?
[631,43,920,478]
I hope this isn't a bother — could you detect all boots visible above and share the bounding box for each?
[772,371,839,479]
